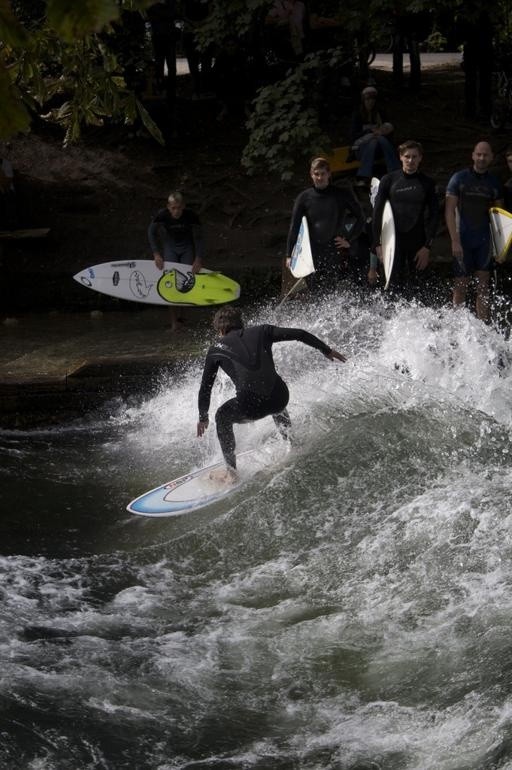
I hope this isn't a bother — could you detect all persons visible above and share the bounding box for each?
[148,192,205,334]
[197,305,343,467]
[353,87,399,186]
[286,157,366,303]
[368,140,512,327]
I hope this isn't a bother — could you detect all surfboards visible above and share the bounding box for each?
[488,204,512,263]
[288,216,317,278]
[73,260,241,307]
[126,441,289,517]
[370,175,396,292]
